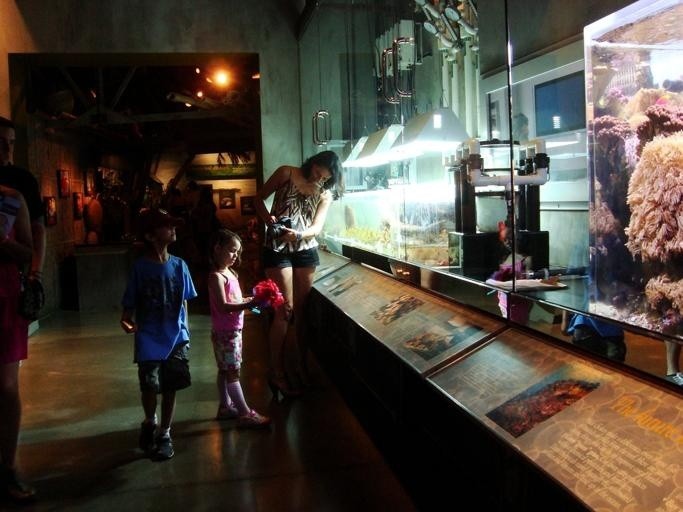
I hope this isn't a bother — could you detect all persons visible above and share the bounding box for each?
[490,227,535,282]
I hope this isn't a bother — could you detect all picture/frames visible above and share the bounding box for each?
[41,166,260,230]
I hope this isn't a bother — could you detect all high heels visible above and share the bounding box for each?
[267,378,303,402]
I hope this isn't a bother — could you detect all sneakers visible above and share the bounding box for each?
[148,433,175,461]
[237,408,273,429]
[0,480,41,505]
[215,403,238,421]
[137,419,156,449]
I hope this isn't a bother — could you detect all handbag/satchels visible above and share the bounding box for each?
[260,214,296,267]
[19,277,45,323]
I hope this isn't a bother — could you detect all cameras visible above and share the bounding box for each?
[267,216,292,239]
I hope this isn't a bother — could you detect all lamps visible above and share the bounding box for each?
[336,133,367,166]
[354,125,404,166]
[389,90,475,159]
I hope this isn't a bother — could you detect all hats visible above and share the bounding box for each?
[138,207,186,229]
